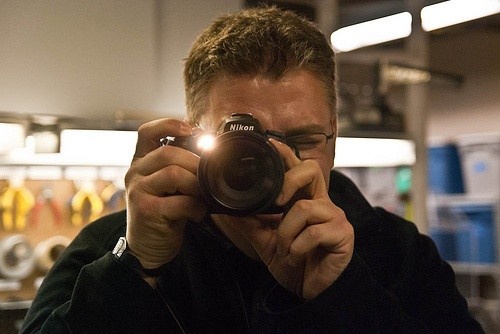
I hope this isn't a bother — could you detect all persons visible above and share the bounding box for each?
[19,8,486,334]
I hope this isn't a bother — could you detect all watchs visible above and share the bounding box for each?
[110,235,165,276]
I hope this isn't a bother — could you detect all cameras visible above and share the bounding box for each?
[173,112,300,216]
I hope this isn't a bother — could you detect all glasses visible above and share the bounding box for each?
[284,131,337,159]
